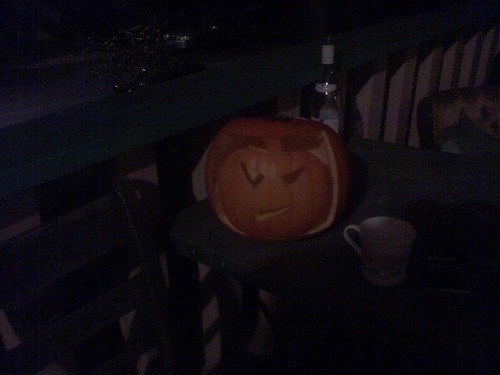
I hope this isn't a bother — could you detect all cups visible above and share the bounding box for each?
[344,216,416,285]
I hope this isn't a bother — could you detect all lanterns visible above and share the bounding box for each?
[204,116,351,241]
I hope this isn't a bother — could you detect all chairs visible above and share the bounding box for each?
[0,186,177,375]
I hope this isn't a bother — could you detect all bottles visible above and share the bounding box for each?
[312,36,343,132]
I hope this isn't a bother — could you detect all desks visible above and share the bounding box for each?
[171,136,500,375]
